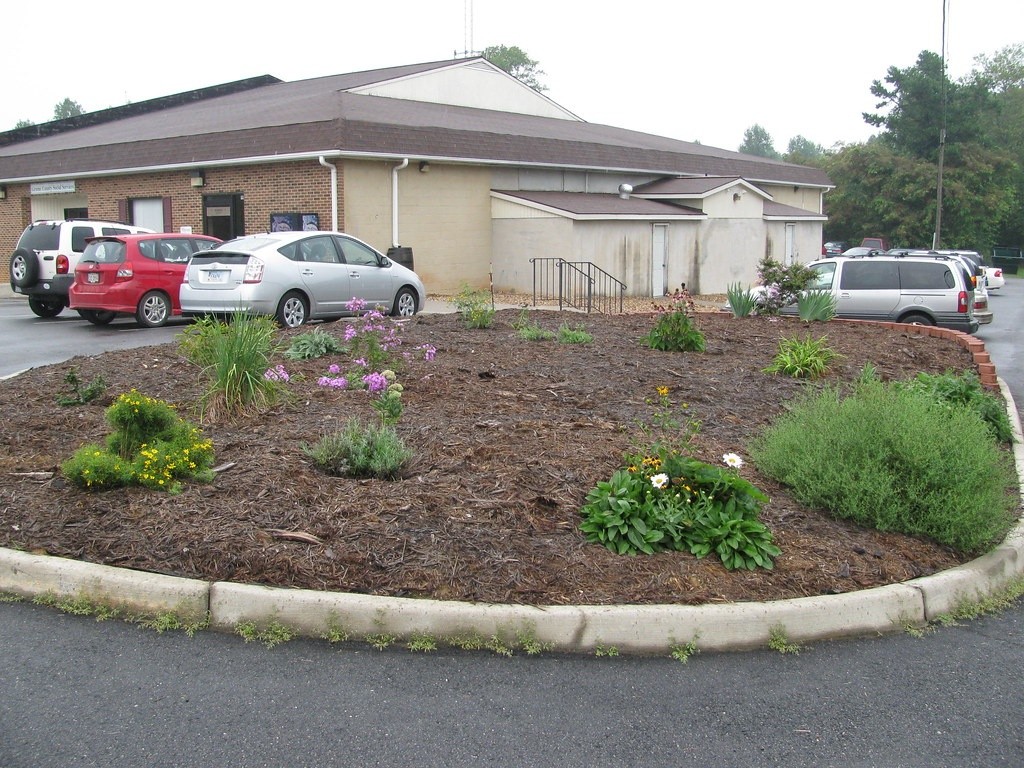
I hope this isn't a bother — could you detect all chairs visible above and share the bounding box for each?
[160,244,170,258]
[306,244,328,262]
[173,244,192,258]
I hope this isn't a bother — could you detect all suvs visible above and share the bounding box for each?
[743,246,1005,335]
[8,217,178,318]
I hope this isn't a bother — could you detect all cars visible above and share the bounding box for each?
[179,230,426,330]
[822,241,853,259]
[69,232,225,327]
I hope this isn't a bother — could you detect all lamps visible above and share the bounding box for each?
[419,161,431,172]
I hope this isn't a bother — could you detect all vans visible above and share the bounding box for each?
[860,237,888,253]
